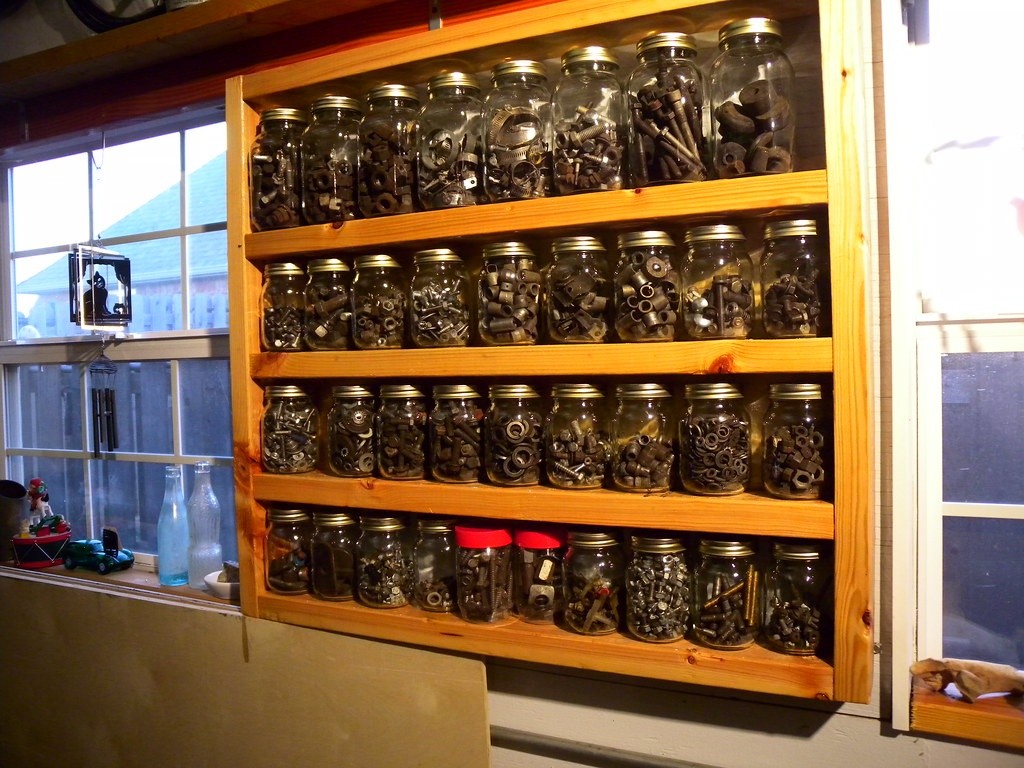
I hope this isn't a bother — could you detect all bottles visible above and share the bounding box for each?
[156,467,191,587]
[187,462,224,591]
[249,19,828,658]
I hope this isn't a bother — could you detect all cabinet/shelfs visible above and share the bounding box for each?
[226,0,872,703]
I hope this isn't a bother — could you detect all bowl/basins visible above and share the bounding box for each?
[203,571,240,599]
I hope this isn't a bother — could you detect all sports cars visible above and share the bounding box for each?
[62,539,135,575]
